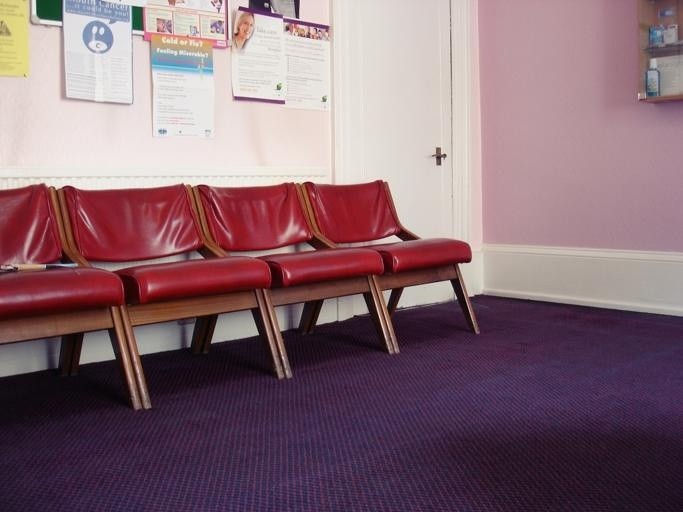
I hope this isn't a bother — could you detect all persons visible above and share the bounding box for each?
[233,12,255,50]
[286,22,326,41]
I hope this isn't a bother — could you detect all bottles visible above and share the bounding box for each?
[645,9,679,97]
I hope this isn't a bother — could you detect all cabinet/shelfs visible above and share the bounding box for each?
[636,0,683,103]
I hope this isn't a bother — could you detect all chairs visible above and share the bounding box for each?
[193,182,395,380]
[0,183,143,411]
[302,180,481,355]
[56,184,286,411]
